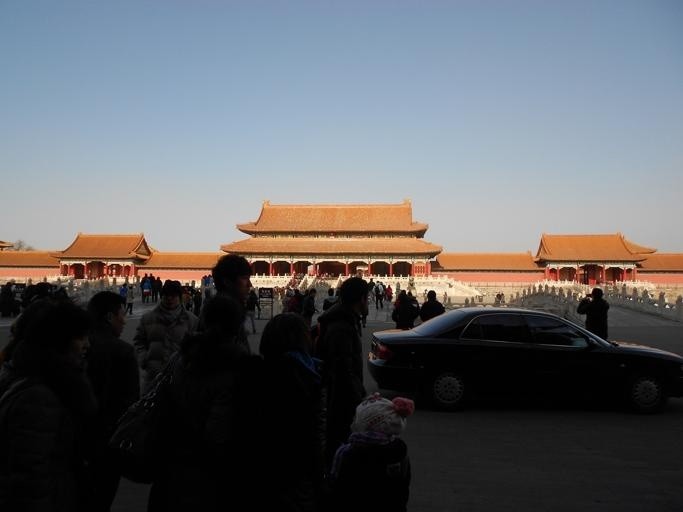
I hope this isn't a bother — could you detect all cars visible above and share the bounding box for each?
[366,306,683,413]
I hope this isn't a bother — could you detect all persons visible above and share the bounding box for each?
[576,287,610,340]
[495,293,505,305]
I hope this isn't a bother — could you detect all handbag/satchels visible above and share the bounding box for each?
[108,387,171,484]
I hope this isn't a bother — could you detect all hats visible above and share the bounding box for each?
[160,279,183,298]
[355,391,416,433]
